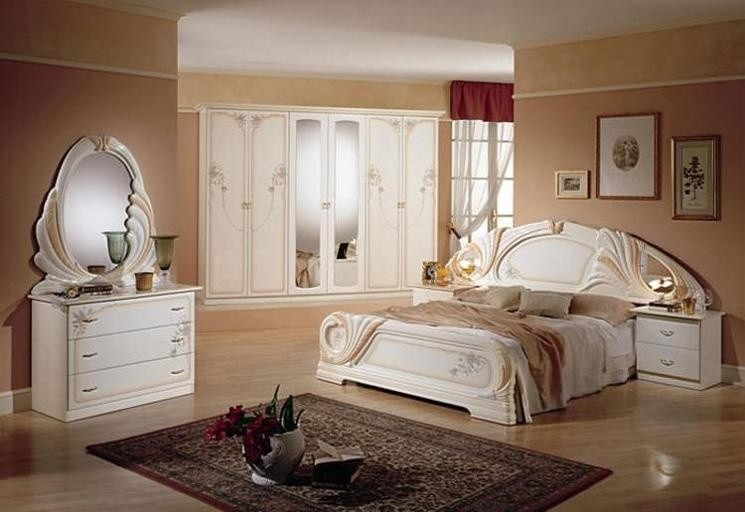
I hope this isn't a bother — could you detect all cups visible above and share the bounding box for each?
[683,297,696,314]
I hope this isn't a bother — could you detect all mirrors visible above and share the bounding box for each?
[29,133,160,295]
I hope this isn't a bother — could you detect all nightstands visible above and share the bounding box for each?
[629,298,727,390]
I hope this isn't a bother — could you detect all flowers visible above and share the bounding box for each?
[203,383,304,461]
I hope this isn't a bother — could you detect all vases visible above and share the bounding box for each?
[240,425,304,486]
[134,234,180,291]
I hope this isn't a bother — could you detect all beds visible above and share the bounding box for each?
[315,220,705,426]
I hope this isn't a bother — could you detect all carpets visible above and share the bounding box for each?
[86,392,613,510]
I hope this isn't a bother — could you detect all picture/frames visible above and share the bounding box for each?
[555,111,722,222]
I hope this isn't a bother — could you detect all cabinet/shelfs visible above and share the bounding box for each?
[26,278,203,424]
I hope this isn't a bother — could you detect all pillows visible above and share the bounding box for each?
[453,285,638,327]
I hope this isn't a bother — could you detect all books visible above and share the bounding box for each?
[311,439,365,484]
[71,284,113,292]
[648,306,682,313]
[80,290,113,297]
[650,300,682,307]
